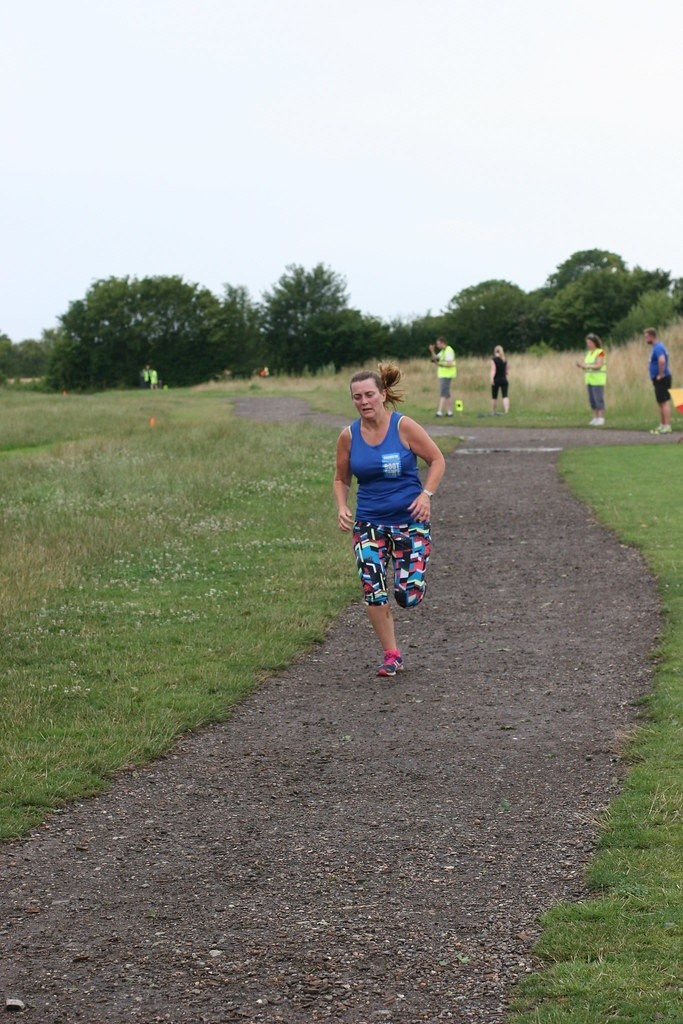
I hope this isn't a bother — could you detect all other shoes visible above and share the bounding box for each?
[436,413,452,417]
[488,412,506,416]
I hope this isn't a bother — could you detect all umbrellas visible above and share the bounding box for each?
[667,388,683,413]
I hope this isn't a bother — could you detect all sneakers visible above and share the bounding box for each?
[374,650,404,676]
[649,424,671,434]
[590,417,605,425]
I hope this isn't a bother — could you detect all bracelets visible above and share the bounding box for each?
[424,490,434,500]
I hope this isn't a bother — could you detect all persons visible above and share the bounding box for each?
[141,365,159,390]
[428,337,457,417]
[643,328,673,435]
[576,333,607,426]
[333,362,445,677]
[490,345,510,415]
